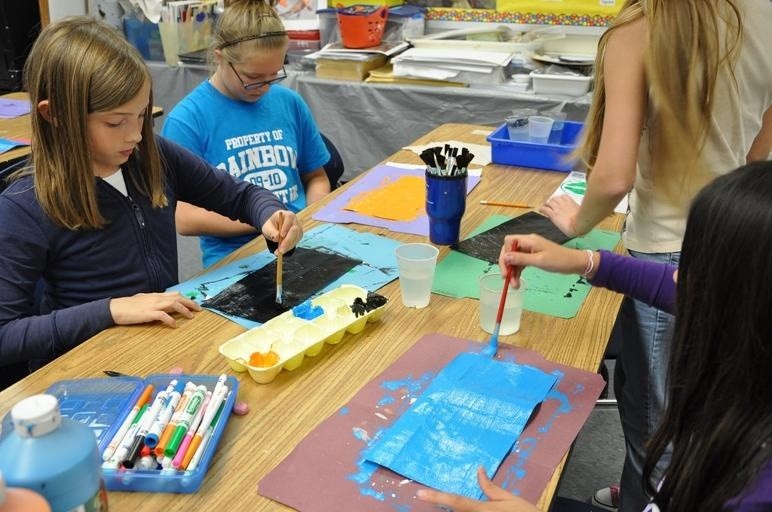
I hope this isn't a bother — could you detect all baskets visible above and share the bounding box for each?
[336,3,388,48]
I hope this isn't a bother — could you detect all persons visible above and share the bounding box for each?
[414,157,772,512]
[1,14,304,389]
[541,0,772,512]
[158,0,337,275]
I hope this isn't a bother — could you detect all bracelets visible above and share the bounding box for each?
[579,246,595,277]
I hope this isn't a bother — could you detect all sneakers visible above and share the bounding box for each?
[591,487,623,511]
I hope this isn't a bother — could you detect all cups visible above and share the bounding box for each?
[394,242,440,309]
[477,273,527,336]
[425,163,468,246]
[504,107,568,145]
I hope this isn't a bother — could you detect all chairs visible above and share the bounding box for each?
[0,157,34,193]
[318,132,344,192]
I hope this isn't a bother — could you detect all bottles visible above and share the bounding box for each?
[0,394,109,512]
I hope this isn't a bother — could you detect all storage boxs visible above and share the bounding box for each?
[316,4,426,50]
[486,119,585,172]
[529,70,594,97]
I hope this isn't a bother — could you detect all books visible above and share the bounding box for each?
[159,0,218,55]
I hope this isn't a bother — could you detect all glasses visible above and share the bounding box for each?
[228,61,287,90]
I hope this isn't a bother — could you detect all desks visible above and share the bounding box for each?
[0,92,164,175]
[0,123,626,512]
[141,58,592,283]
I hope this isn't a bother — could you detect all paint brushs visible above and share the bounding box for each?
[275,212,284,304]
[481,240,518,358]
[420,144,474,176]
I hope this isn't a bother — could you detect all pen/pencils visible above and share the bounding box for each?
[104,370,129,376]
[102,375,233,491]
[480,201,535,209]
